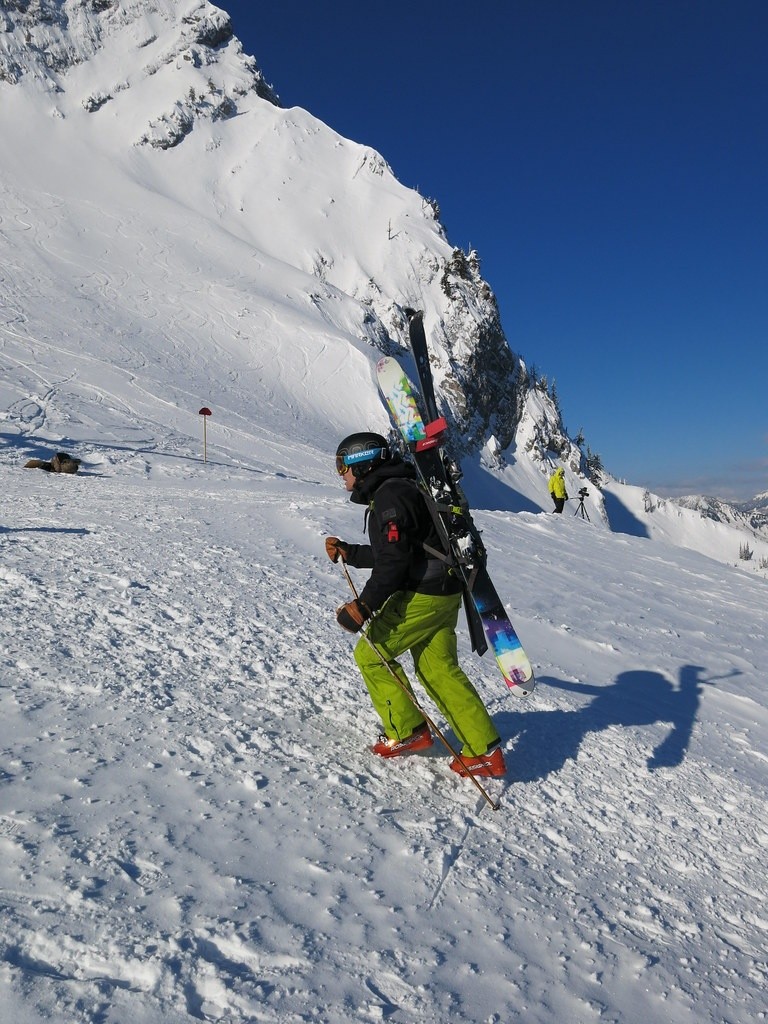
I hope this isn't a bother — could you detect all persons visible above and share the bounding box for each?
[548,467,568,514]
[325,431,509,777]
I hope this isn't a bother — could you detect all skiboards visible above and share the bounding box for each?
[375,306,540,701]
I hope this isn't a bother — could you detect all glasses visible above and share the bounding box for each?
[335,448,384,475]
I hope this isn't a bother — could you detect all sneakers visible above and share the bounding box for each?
[449,747,507,777]
[373,721,434,757]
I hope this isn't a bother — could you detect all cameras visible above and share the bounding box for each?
[579,487,589,497]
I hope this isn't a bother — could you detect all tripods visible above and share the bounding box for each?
[570,497,590,523]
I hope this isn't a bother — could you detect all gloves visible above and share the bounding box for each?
[551,492,557,500]
[565,493,568,501]
[325,537,350,564]
[336,598,373,635]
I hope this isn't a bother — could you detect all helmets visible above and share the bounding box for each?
[336,432,391,476]
